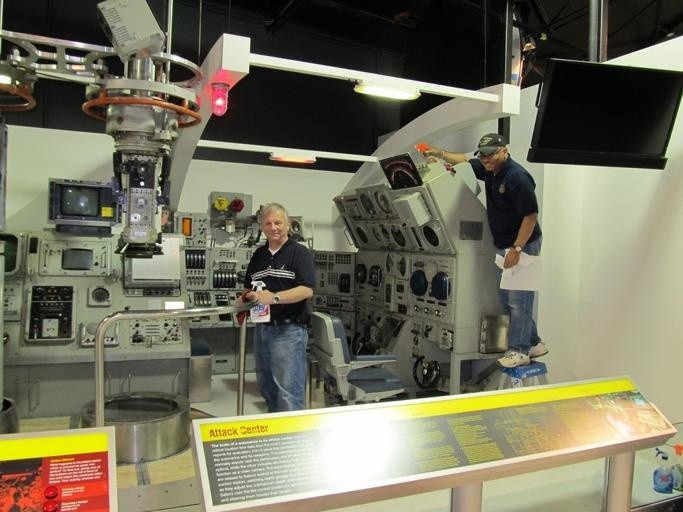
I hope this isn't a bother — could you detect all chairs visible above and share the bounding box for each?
[311,311,405,406]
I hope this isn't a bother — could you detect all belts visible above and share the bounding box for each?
[262,319,294,326]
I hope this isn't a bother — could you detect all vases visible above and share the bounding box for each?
[225,217,235,234]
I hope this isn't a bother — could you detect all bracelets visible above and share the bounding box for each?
[441,150,444,158]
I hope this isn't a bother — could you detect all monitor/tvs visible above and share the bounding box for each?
[49,178,119,227]
[526,57,683,170]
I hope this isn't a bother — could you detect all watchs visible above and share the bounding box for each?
[272,291,280,305]
[512,245,522,254]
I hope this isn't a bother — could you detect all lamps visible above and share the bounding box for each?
[212,83,229,116]
[269,151,317,164]
[353,79,421,100]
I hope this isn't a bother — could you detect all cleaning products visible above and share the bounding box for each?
[249,280,271,325]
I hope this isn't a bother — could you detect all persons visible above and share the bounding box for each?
[420,132,548,369]
[235,202,315,413]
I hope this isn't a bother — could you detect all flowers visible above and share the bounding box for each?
[214,196,244,218]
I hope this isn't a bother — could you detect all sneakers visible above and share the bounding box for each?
[497,350,532,368]
[529,340,549,360]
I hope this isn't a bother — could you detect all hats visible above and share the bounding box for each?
[474,134,507,156]
